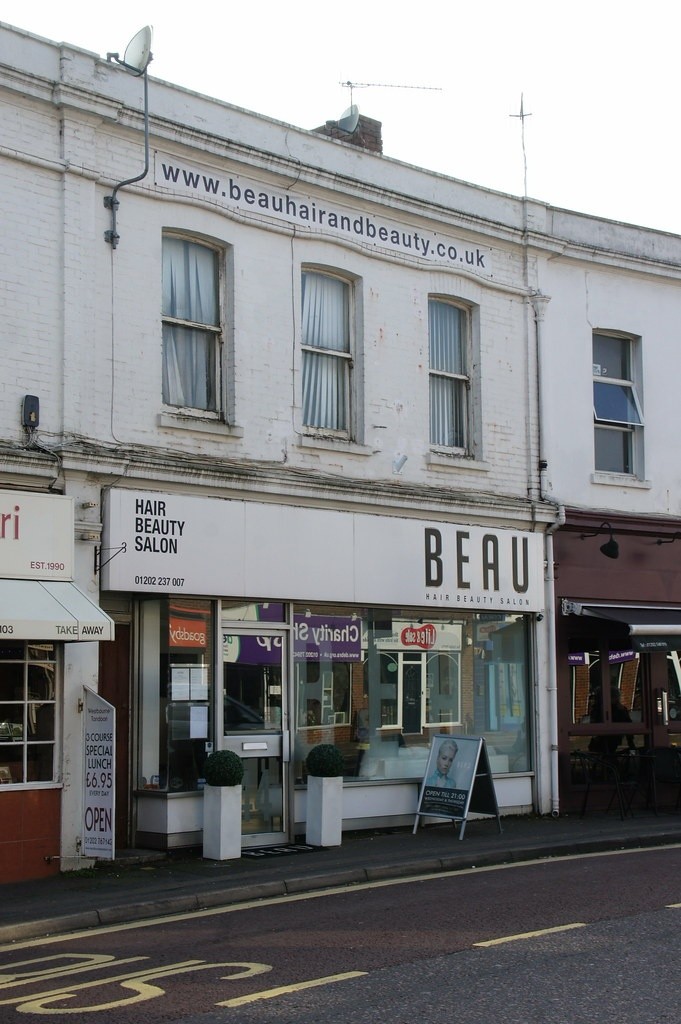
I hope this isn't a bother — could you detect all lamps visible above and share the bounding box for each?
[417,617,423,624]
[330,613,357,621]
[294,609,311,618]
[448,616,455,625]
[580,521,619,560]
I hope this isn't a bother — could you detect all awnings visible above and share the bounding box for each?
[585,605,681,652]
[0,577,115,643]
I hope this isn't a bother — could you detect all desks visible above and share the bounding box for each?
[588,753,657,817]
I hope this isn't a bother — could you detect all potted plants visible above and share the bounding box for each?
[202,750,242,860]
[306,742,344,846]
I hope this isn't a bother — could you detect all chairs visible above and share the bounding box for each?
[571,746,680,821]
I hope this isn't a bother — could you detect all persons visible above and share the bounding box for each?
[426,740,459,788]
[588,687,636,754]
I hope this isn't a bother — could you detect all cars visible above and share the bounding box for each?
[163,683,304,779]
